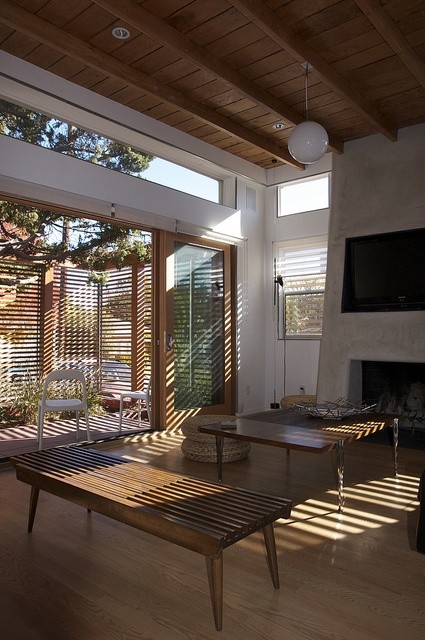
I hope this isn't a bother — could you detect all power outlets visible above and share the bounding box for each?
[298,384,305,394]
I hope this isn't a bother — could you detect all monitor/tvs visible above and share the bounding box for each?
[341,227,423,313]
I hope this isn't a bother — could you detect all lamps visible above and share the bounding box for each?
[288,61,329,164]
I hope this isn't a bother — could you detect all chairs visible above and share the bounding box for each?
[119,376,151,430]
[37,370,91,450]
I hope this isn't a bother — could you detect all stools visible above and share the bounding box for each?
[181,415,251,462]
[280,395,316,410]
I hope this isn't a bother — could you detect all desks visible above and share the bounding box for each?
[198,415,400,513]
[9,446,292,631]
